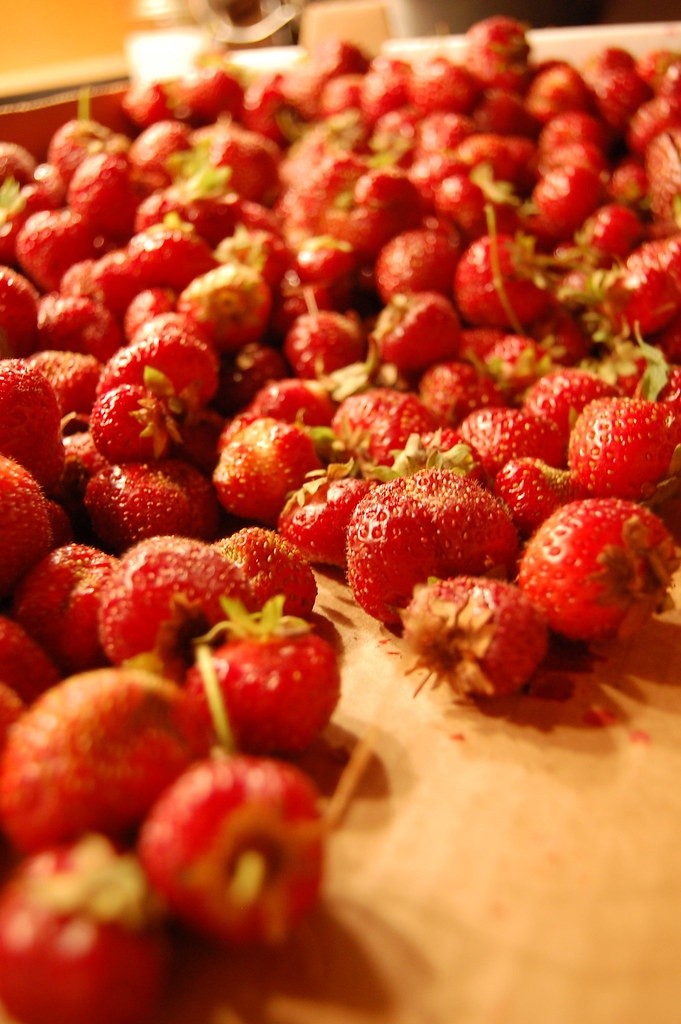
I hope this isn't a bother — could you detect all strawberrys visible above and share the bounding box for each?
[0,16,681,1023]
